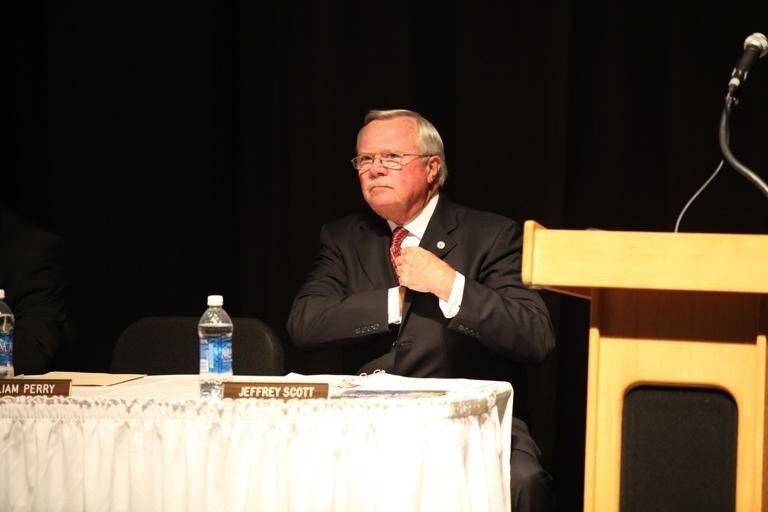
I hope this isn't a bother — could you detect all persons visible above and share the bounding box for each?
[285,107,558,512]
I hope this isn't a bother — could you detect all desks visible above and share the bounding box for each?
[0,369,514,512]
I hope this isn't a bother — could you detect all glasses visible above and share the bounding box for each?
[351,153,433,169]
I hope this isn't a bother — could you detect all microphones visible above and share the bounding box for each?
[716,33,768,196]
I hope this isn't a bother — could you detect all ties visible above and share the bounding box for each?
[390,227,408,286]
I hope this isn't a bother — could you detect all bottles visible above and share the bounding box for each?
[0,289,14,379]
[198,295,233,399]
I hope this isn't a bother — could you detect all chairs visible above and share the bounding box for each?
[110,315,284,374]
